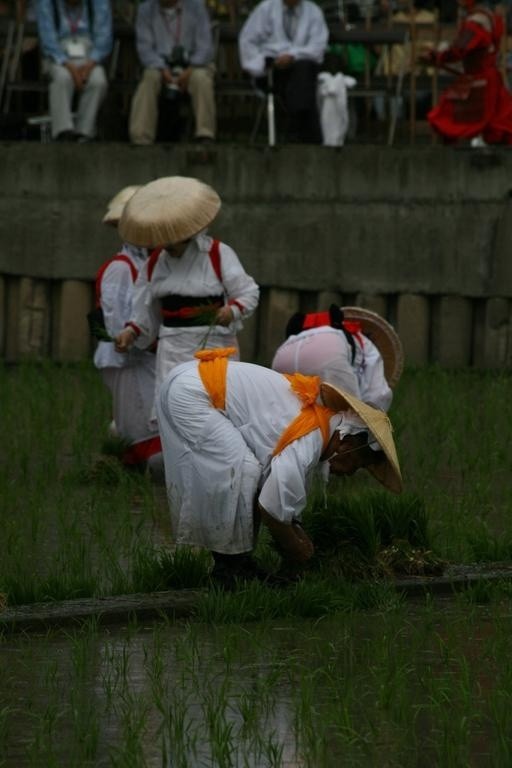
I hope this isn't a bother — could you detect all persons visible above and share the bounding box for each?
[266,309,395,413]
[29,0,512,152]
[113,239,261,395]
[93,241,161,474]
[153,348,383,582]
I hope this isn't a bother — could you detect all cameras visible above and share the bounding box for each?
[164,46,190,76]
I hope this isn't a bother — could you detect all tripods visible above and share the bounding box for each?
[250,71,287,146]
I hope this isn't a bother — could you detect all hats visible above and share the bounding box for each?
[339,307,405,388]
[118,175,222,247]
[102,185,143,227]
[320,382,403,494]
